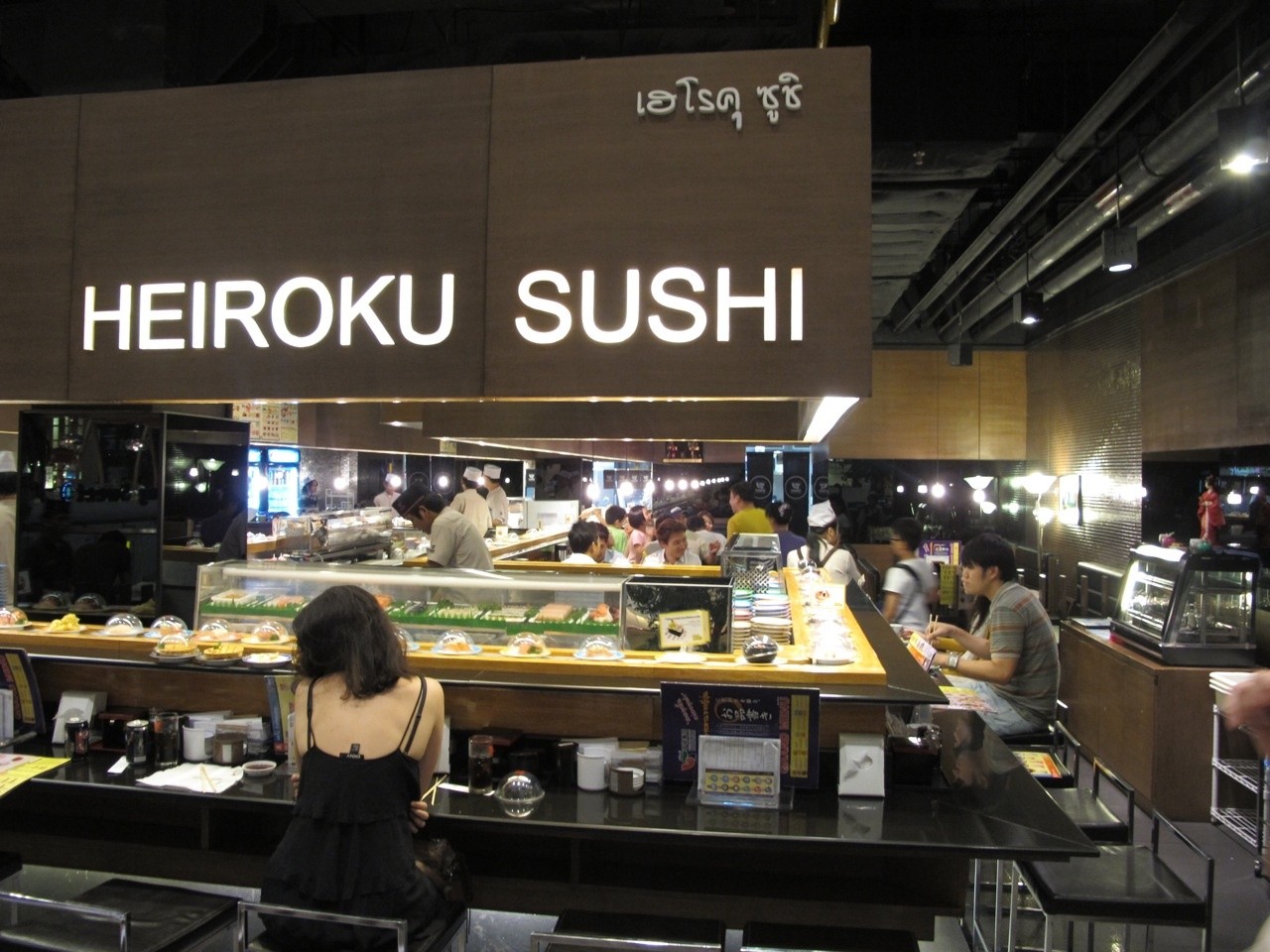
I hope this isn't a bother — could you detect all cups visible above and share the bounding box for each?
[467,733,494,794]
[577,743,612,791]
[153,711,181,769]
[213,737,248,764]
[183,722,213,762]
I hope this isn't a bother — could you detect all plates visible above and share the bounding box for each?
[150,650,198,664]
[0,621,33,629]
[499,647,552,659]
[572,651,625,662]
[241,634,293,645]
[402,640,421,653]
[45,625,86,633]
[614,764,645,795]
[428,645,483,655]
[242,759,277,777]
[143,630,194,640]
[723,580,794,657]
[102,626,148,638]
[197,653,242,666]
[241,651,293,669]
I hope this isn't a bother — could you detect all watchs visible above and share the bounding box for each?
[948,655,959,675]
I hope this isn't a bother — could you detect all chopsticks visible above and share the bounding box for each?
[419,774,448,808]
[927,612,939,648]
[199,763,218,795]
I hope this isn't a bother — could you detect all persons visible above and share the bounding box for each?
[923,533,1061,738]
[830,494,883,544]
[786,499,861,584]
[219,509,247,560]
[765,500,806,567]
[297,474,319,516]
[949,711,1020,813]
[450,467,492,537]
[0,451,18,607]
[256,583,464,952]
[726,481,774,541]
[557,487,730,566]
[392,480,502,605]
[73,530,131,583]
[900,593,992,662]
[483,465,509,538]
[881,517,938,630]
[374,472,402,507]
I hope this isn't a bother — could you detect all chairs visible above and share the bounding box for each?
[745,918,921,952]
[0,873,243,952]
[970,756,1135,951]
[1010,719,1082,796]
[230,897,473,952]
[1010,805,1216,952]
[532,908,727,952]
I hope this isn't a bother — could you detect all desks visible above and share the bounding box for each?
[1054,621,1270,825]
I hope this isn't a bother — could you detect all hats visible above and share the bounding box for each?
[391,481,430,518]
[483,464,501,479]
[303,474,316,485]
[385,473,401,487]
[806,501,836,527]
[463,466,482,481]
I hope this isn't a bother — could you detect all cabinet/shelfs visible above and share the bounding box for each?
[1106,535,1262,667]
[1210,704,1270,880]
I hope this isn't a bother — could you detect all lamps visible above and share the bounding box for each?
[924,353,1033,496]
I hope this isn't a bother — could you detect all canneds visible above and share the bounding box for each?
[63,716,91,761]
[124,720,154,771]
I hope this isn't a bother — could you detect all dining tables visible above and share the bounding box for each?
[8,722,1097,952]
